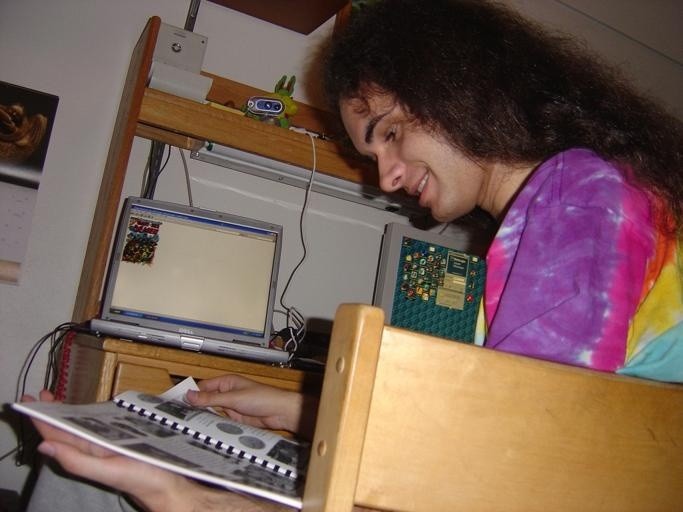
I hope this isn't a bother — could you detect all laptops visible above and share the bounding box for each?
[90,195,290,365]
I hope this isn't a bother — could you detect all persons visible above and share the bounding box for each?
[20,0,683,512]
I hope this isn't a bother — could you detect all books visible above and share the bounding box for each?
[10,374,311,509]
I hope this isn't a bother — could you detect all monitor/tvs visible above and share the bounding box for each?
[373,221,487,347]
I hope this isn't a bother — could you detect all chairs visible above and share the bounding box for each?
[297,301,683,511]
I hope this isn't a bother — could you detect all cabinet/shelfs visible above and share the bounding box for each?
[53,10,412,422]
